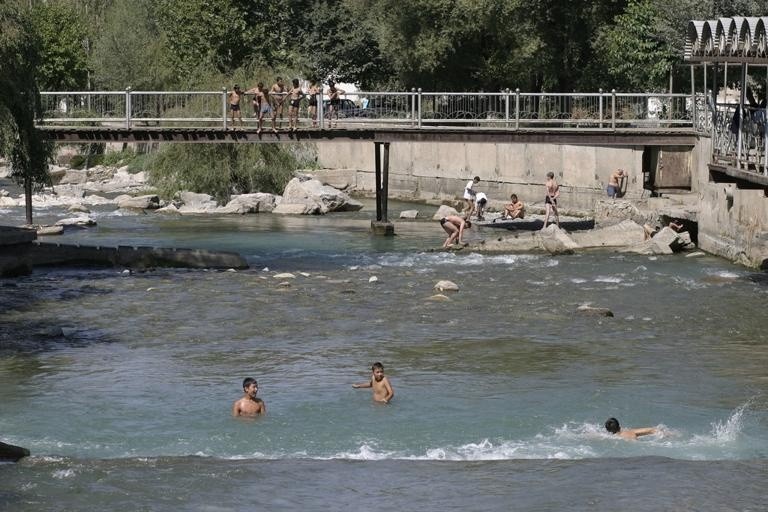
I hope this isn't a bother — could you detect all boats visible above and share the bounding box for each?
[480,220,595,232]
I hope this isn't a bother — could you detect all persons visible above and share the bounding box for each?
[227,76,345,134]
[502,193,524,220]
[670,219,685,233]
[475,192,488,220]
[606,168,627,198]
[353,363,393,404]
[540,171,561,229]
[439,215,471,247]
[604,417,656,439]
[461,176,480,222]
[232,377,266,417]
